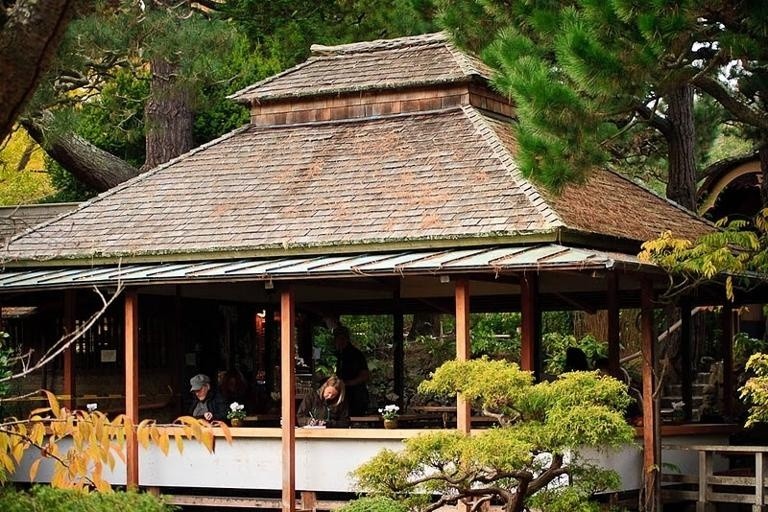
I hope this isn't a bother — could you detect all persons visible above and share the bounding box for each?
[188,373,230,421]
[566,348,588,373]
[296,376,349,429]
[332,325,369,417]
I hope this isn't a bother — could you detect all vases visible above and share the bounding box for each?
[231,418,240,427]
[271,406,280,416]
[673,414,686,425]
[384,420,397,429]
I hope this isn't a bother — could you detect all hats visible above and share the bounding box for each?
[190,374,210,391]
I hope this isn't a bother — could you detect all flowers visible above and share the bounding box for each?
[227,401,246,420]
[270,392,281,402]
[378,404,400,420]
[671,401,686,413]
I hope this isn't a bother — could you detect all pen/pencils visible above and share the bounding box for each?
[309,411,314,419]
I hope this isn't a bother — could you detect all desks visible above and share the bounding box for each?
[425,406,457,429]
[237,415,498,421]
